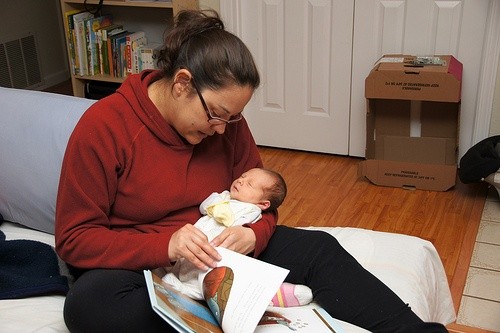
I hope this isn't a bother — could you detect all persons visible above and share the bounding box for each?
[54,9,449,333]
[194,167,287,242]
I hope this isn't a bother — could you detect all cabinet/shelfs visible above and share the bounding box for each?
[60,0,199,100]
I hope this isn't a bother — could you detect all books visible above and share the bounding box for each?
[61,6,166,78]
[144,245,342,333]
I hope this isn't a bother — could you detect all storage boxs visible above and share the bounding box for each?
[357,55,463,191]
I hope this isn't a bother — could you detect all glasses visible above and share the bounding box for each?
[180,65,244,123]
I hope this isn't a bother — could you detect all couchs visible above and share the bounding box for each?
[0,87,457,333]
